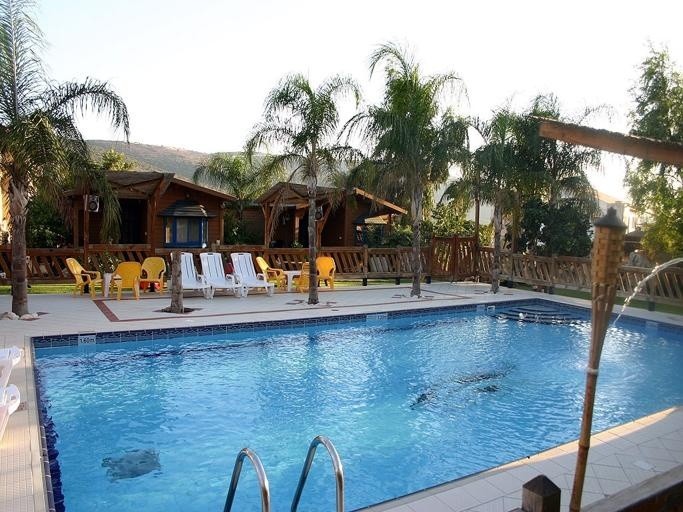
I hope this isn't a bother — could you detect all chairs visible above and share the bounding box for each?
[293,256,336,293]
[110,260,142,302]
[169,251,276,299]
[255,255,286,291]
[65,256,100,299]
[140,256,166,297]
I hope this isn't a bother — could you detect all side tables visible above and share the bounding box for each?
[102,272,121,298]
[282,270,300,293]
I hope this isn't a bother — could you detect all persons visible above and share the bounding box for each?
[408,373,500,405]
